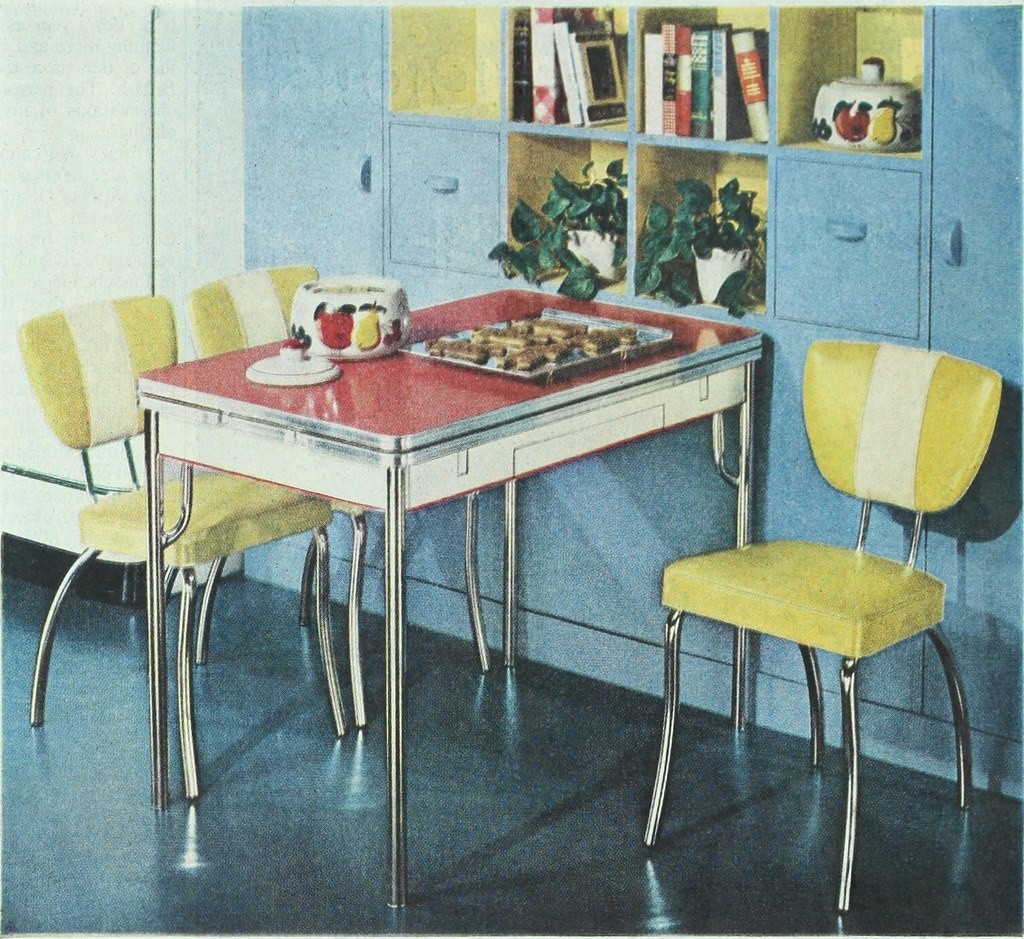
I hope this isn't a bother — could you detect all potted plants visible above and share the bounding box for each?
[488,153,628,302]
[633,173,763,322]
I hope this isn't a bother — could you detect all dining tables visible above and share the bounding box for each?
[132,288,764,913]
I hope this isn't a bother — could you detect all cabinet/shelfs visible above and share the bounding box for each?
[239,8,1021,808]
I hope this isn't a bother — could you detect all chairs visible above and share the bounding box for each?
[640,337,1008,910]
[14,294,350,744]
[181,262,492,726]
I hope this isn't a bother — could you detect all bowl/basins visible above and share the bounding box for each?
[290,277,411,361]
[813,59,921,152]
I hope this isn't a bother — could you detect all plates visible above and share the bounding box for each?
[397,307,674,383]
